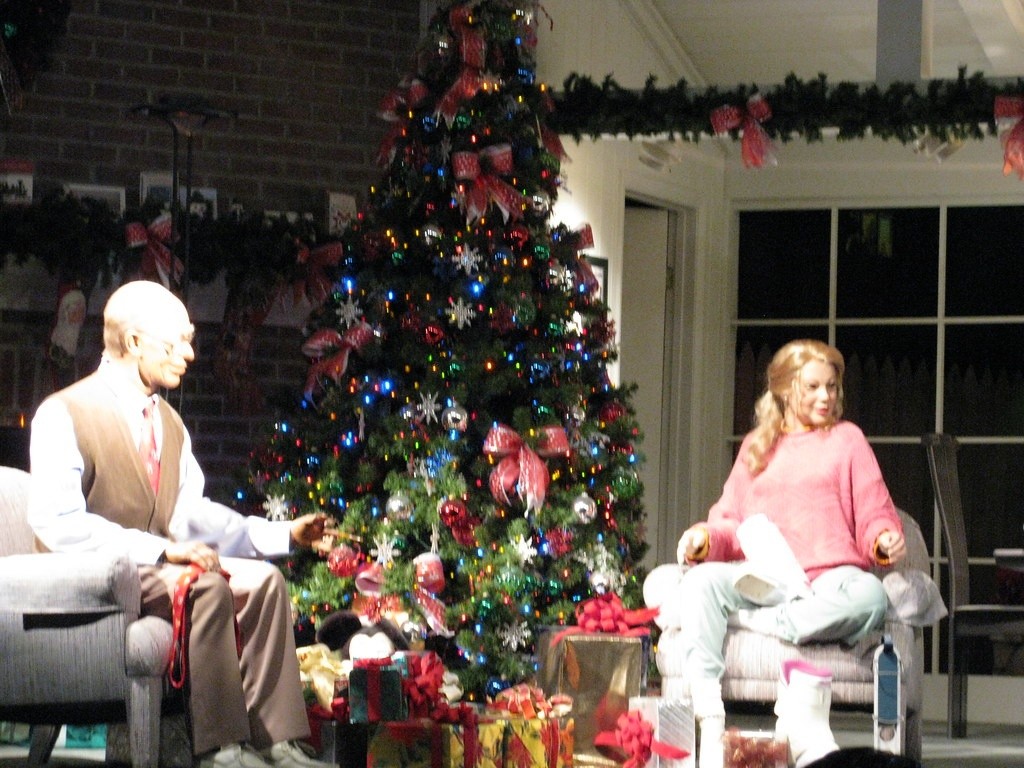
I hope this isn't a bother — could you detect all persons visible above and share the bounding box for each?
[27,280,334,768]
[677,340,905,768]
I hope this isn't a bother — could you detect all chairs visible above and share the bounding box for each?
[920,431,1024,743]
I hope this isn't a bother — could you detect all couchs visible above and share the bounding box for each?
[0,466,187,768]
[645,505,948,760]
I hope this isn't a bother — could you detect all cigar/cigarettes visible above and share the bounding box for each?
[324,526,363,543]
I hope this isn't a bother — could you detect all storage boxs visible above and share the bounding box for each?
[331,720,442,767]
[539,626,643,755]
[448,719,575,768]
[346,654,411,719]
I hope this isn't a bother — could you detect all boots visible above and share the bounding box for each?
[691,677,726,768]
[770,661,840,767]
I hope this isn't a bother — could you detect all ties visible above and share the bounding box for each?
[139,406,160,498]
[167,562,243,689]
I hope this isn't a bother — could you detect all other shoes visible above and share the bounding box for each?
[270,740,339,768]
[201,742,276,768]
